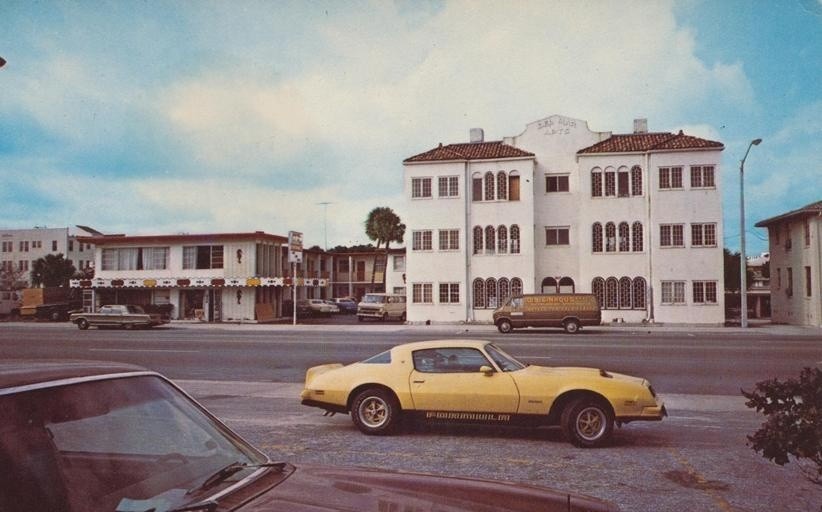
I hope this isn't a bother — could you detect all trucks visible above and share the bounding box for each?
[17,287,82,320]
[492,293,601,333]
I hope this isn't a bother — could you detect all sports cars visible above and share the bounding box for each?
[301,339,669,449]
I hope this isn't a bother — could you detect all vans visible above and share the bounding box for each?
[357,293,406,321]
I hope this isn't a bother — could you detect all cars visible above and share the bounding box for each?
[70,304,170,330]
[0,359,623,512]
[283,297,357,316]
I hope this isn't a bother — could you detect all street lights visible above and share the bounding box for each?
[740,138,763,328]
